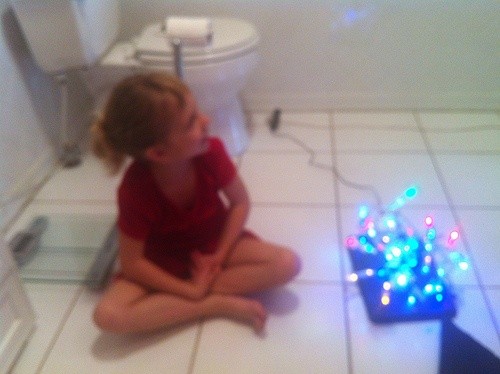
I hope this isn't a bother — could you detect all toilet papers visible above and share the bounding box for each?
[164,17,212,46]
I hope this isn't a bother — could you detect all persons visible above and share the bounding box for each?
[82,70,304,339]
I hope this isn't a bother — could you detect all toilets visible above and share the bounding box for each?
[9,0,262,157]
